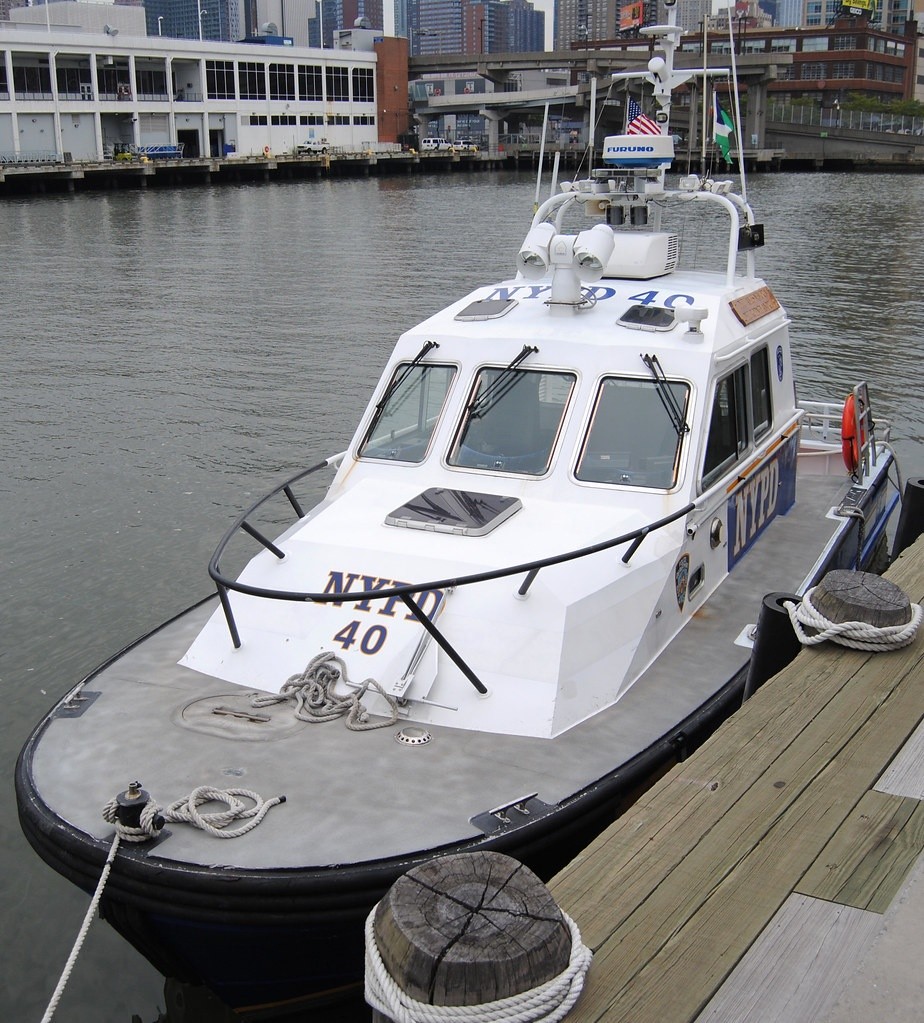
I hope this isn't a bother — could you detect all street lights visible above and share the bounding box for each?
[480,18,487,54]
[697,20,704,57]
[158,16,163,36]
[198,9,207,41]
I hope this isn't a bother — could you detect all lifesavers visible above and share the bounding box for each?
[265,147,269,152]
[841,392,865,472]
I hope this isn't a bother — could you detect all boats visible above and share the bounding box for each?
[13,0,902,1010]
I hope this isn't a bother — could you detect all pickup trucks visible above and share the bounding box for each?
[298,138,330,155]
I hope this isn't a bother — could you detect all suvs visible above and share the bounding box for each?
[453,140,478,152]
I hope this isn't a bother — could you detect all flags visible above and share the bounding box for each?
[626,96,661,135]
[712,91,734,164]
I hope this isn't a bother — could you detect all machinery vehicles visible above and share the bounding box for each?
[111,142,131,161]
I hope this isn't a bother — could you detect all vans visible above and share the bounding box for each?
[422,138,451,151]
